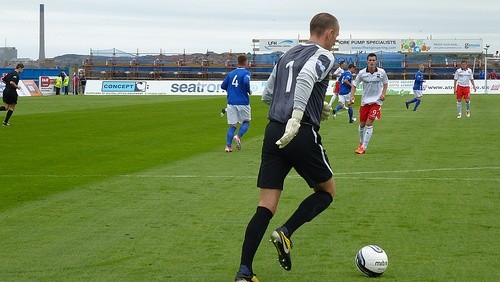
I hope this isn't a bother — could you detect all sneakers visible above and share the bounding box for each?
[343,106,348,110]
[349,118,356,123]
[2,121,10,126]
[466,110,470,117]
[333,108,336,119]
[221,109,225,117]
[405,101,409,109]
[234,271,259,282]
[355,145,366,154]
[328,104,333,111]
[457,113,461,118]
[358,143,362,148]
[269,227,293,271]
[225,146,233,152]
[233,135,241,150]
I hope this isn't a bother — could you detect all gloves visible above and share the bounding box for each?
[275,119,301,148]
[321,101,331,121]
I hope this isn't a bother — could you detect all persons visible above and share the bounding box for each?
[80,76,86,93]
[328,61,349,110]
[454,60,476,119]
[235,13,339,282]
[333,64,356,123]
[55,75,61,95]
[73,74,79,95]
[0,64,24,126]
[480,70,485,80]
[406,64,424,111]
[351,53,388,154]
[221,55,252,152]
[64,74,69,95]
[489,71,496,79]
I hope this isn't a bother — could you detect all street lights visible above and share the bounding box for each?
[483,43,490,90]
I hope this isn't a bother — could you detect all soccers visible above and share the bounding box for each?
[355,245,389,277]
